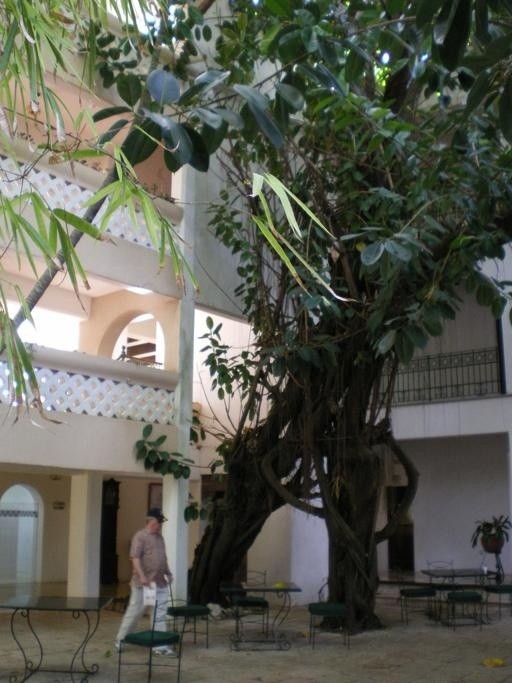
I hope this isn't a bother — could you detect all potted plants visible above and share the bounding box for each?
[470,514,512,554]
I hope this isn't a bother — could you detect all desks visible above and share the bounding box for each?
[1,596,114,682]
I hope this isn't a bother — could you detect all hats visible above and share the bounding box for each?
[145,509,166,524]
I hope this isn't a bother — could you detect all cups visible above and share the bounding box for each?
[483,566,487,574]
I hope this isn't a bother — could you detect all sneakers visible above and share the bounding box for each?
[151,647,178,657]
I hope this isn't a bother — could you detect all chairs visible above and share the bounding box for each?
[118,569,361,683]
[395,559,512,632]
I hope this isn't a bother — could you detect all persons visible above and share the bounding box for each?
[113,508,178,658]
